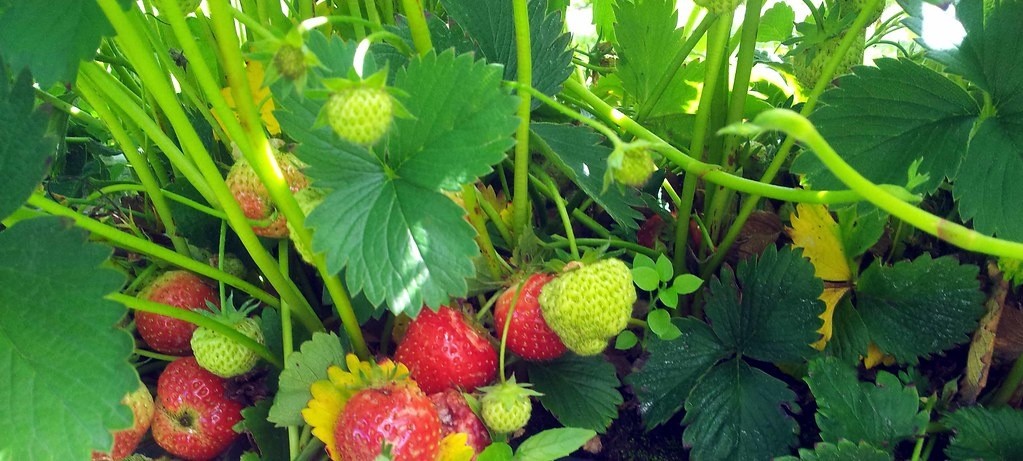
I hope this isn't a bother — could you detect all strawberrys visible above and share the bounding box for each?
[91,46,703,461]
